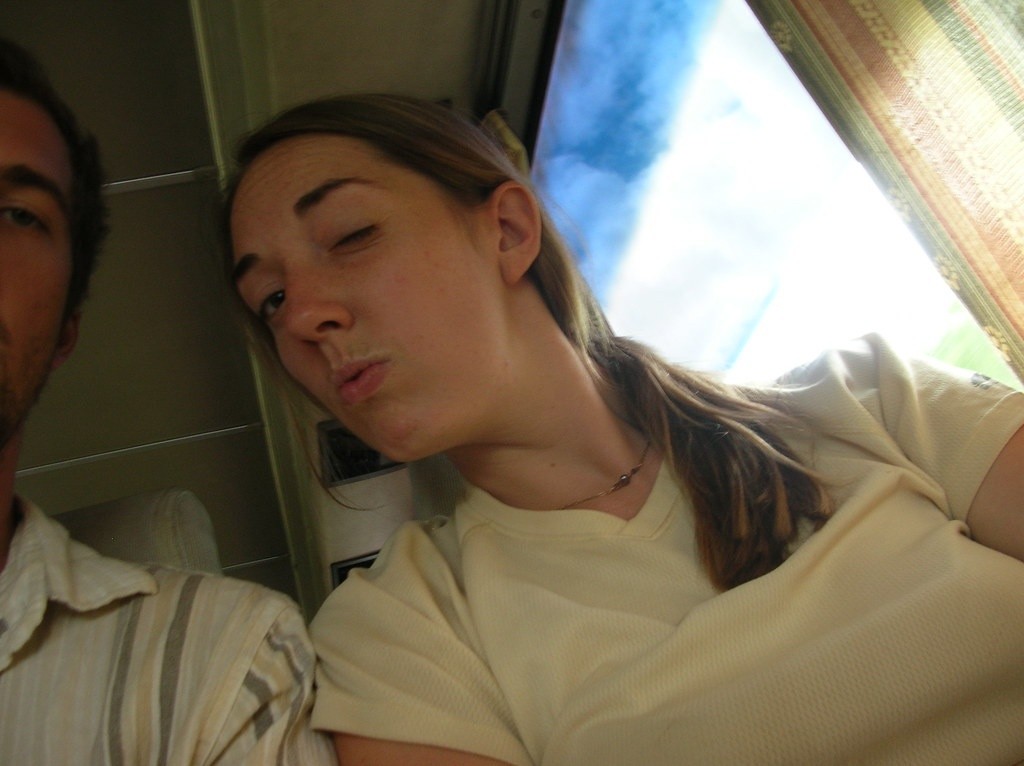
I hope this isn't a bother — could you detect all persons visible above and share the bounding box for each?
[222,92,1024,766]
[0,33,335,766]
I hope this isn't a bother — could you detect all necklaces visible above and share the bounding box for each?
[556,439,651,510]
